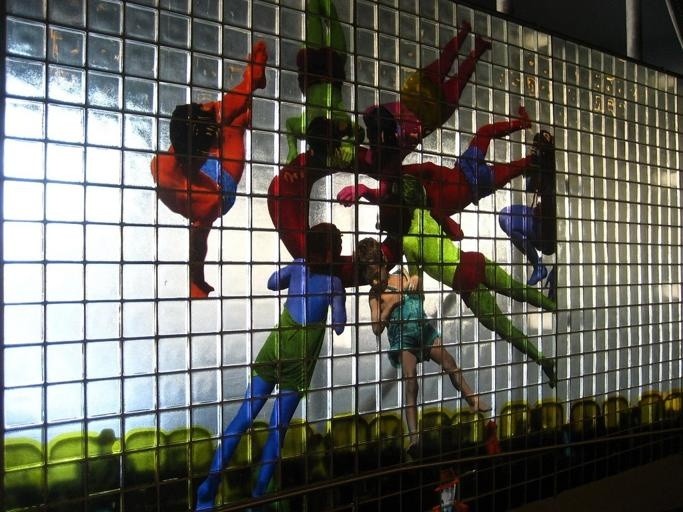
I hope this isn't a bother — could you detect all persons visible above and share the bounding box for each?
[385,106,539,243]
[379,202,557,394]
[193,220,347,511]
[147,41,270,299]
[428,465,467,512]
[496,129,558,306]
[346,240,492,456]
[335,16,493,209]
[282,0,364,180]
